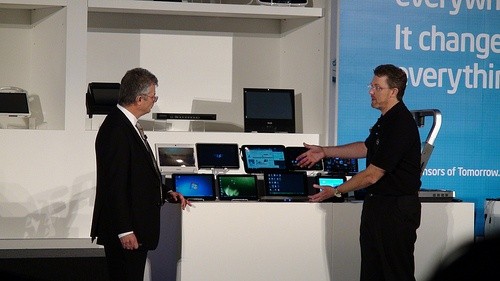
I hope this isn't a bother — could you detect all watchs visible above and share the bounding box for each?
[334,186,343,198]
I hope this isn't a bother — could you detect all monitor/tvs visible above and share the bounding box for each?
[151,143,364,200]
[243,87,295,132]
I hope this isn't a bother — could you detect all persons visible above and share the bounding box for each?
[295,65,422,281]
[91,68,191,281]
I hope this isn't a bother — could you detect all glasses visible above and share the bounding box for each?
[140,93,158,102]
[368,84,400,92]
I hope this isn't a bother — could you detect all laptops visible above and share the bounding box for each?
[0,92,31,117]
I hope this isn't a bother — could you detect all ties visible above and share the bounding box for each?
[135,121,162,199]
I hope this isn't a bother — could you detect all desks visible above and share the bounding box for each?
[143,202,476,281]
